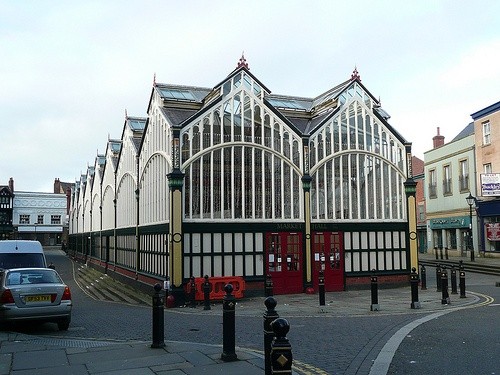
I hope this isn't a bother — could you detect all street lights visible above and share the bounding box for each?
[34,222,38,240]
[465,192,477,264]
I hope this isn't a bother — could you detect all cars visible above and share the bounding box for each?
[0,267,72,331]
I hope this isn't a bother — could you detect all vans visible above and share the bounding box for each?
[0,238,57,284]
